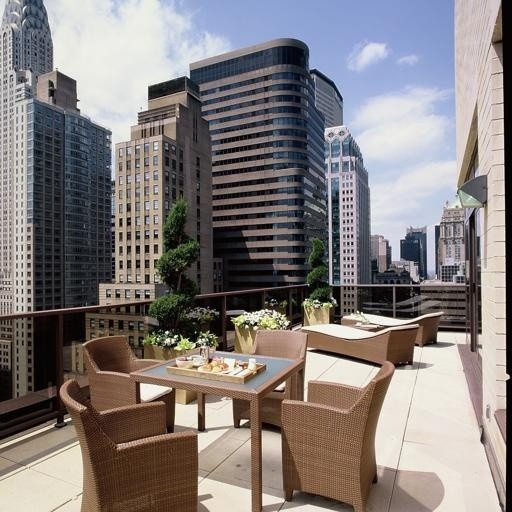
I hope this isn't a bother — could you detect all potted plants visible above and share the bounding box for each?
[141,195,219,405]
[301,237,338,326]
[230,308,291,355]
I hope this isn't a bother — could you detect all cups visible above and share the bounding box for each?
[200,344,215,365]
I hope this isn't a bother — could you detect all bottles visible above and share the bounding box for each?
[249,358,256,371]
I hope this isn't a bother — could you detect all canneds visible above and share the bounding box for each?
[200,346,209,363]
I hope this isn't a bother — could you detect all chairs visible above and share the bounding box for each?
[233,330,308,428]
[59,379,198,512]
[280,361,395,512]
[82,335,176,433]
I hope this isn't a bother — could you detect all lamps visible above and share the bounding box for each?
[456,175,487,208]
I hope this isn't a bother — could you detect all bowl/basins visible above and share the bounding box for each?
[176,355,201,368]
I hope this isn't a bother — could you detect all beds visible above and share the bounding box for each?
[302,323,419,368]
[340,311,445,347]
